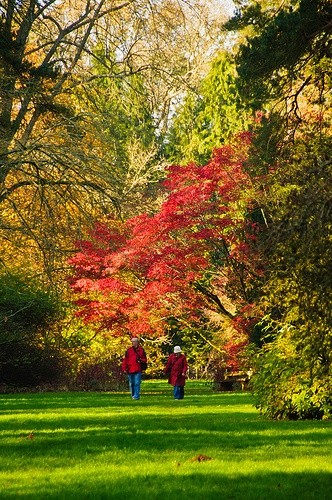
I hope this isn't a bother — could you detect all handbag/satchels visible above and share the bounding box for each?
[167,367,171,377]
[141,362,147,370]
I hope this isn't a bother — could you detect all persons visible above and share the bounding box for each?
[161,346,188,399]
[121,337,147,401]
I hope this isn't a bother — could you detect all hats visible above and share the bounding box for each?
[174,345,182,354]
